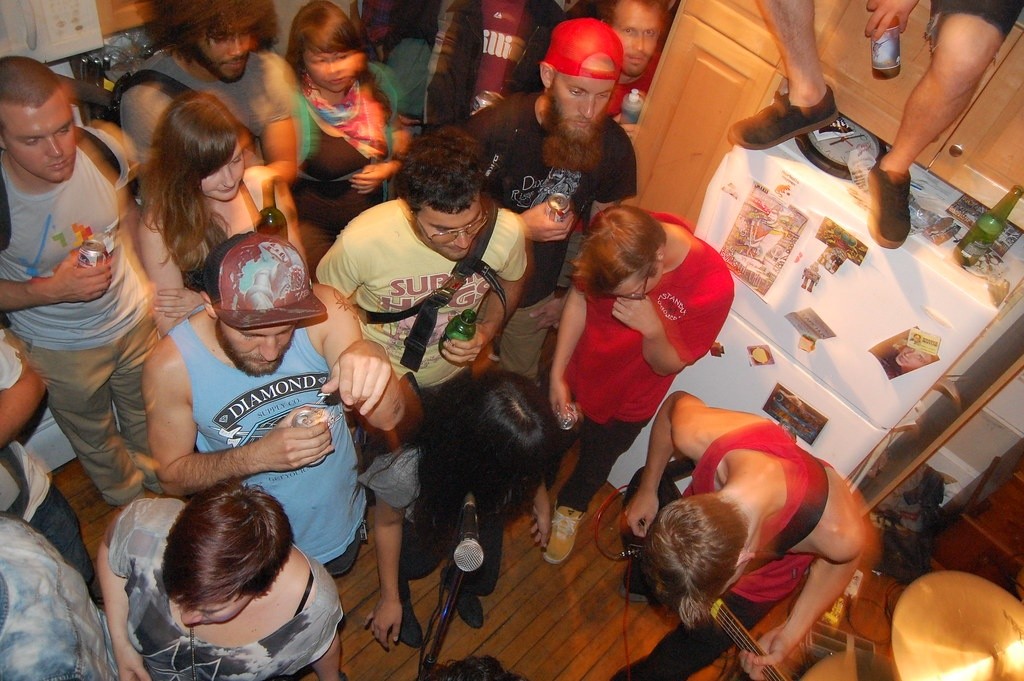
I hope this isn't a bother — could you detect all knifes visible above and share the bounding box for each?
[77,50,112,99]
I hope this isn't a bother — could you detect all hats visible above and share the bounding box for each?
[219,232,327,329]
[538,18,624,79]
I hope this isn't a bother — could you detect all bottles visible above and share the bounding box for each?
[438,309,477,357]
[622,89,646,126]
[870,9,901,79]
[954,185,1023,266]
[254,178,289,248]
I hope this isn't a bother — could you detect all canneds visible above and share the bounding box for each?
[554,404,582,429]
[292,409,326,466]
[544,193,570,222]
[78,240,109,268]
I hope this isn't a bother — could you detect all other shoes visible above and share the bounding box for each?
[398,598,423,648]
[619,559,651,604]
[869,162,910,249]
[457,593,484,629]
[729,84,839,150]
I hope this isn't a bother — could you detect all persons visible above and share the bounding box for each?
[466,17,641,388]
[620,389,869,681]
[598,0,670,139]
[542,203,736,567]
[1,314,119,680]
[348,0,594,129]
[1,53,162,515]
[119,0,304,261]
[315,125,533,449]
[728,0,1024,253]
[357,368,554,650]
[142,233,407,575]
[133,90,308,337]
[413,655,528,681]
[96,483,346,681]
[285,0,413,283]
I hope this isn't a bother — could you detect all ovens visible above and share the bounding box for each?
[0,0,105,70]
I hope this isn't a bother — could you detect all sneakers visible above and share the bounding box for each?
[543,499,585,564]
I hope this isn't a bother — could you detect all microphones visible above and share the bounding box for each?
[453,492,484,572]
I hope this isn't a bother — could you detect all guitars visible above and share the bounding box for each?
[619,464,806,681]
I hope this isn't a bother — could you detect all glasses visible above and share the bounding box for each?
[414,199,489,245]
[622,269,650,300]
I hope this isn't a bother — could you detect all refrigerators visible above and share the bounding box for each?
[594,121,1023,536]
